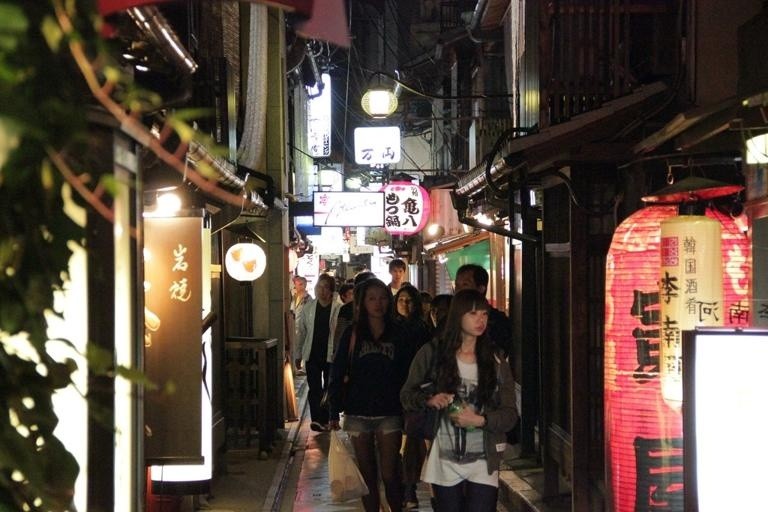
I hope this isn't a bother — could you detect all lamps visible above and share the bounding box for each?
[225,219,266,287]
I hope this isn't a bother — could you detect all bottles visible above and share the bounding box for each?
[447,398,477,433]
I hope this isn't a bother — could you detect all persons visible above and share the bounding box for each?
[291,259,432,431]
[400,289,517,512]
[455,264,512,352]
[326,279,416,512]
[403,294,453,512]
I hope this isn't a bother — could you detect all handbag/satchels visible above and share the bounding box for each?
[403,382,440,440]
[320,358,356,413]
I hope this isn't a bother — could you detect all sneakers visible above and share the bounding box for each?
[402,486,419,509]
[310,422,328,432]
